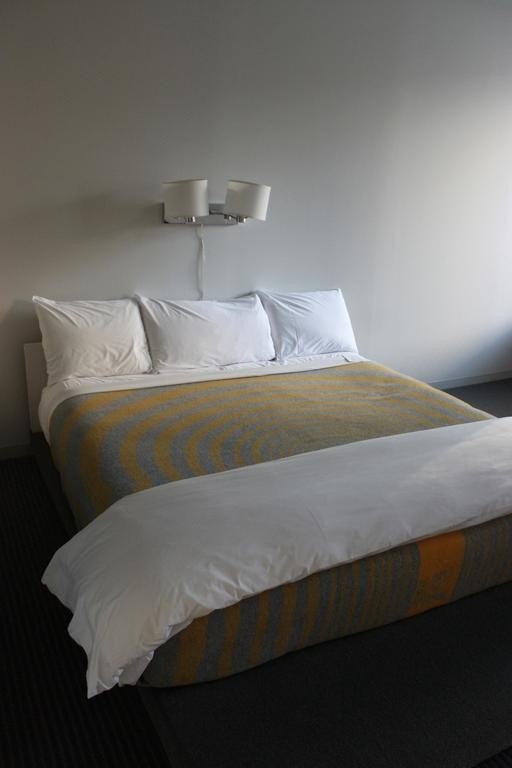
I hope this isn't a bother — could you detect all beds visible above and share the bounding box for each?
[23,343,512,700]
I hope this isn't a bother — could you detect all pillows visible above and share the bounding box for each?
[257,287,359,361]
[135,295,276,375]
[33,296,153,388]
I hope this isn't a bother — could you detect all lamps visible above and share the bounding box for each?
[209,180,272,224]
[162,179,207,225]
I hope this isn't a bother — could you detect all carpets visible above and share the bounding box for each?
[1,434,512,768]
